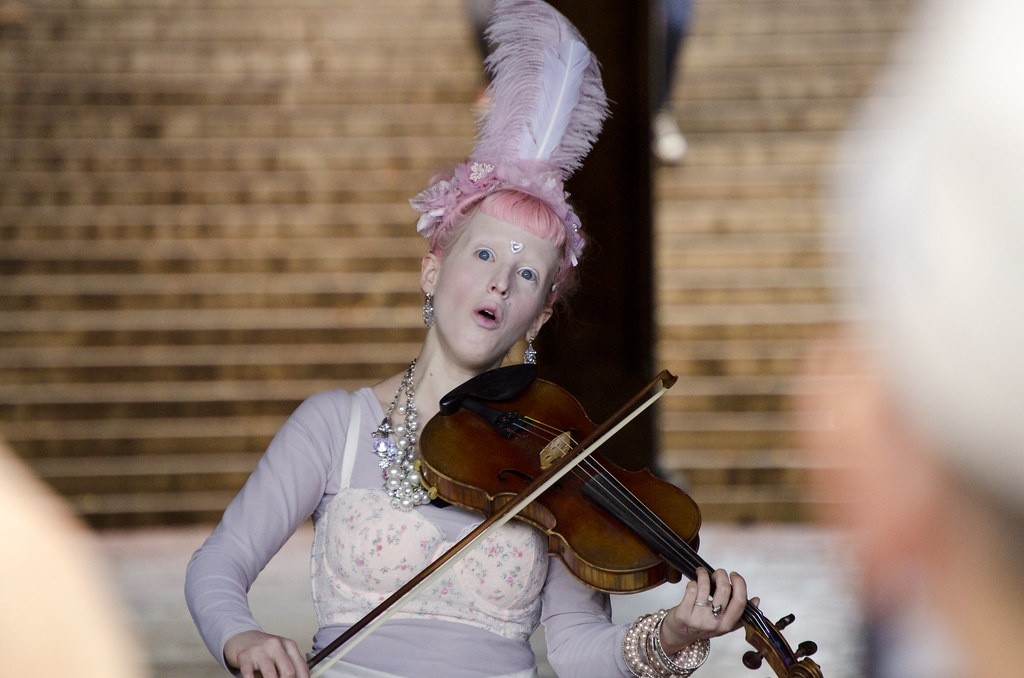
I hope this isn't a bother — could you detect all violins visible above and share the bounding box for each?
[417,361,827,678]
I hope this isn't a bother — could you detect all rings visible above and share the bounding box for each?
[695,600,709,606]
[710,602,726,618]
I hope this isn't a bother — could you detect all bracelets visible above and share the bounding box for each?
[621,608,710,678]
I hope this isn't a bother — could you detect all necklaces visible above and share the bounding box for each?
[369,358,431,511]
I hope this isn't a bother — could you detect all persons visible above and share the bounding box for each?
[649,0,688,161]
[793,1,1023,676]
[176,167,760,678]
[460,0,497,112]
